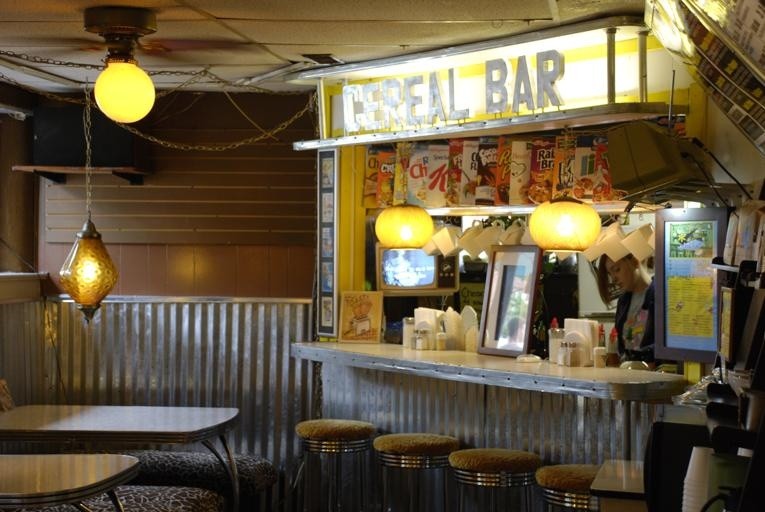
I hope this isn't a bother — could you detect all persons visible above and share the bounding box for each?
[597,251,654,366]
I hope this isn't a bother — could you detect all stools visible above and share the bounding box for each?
[0,485,224,512]
[448,448,539,512]
[536,464,602,512]
[297,419,375,512]
[375,433,460,512]
[59,451,277,512]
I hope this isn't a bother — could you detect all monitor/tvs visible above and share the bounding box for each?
[375,242,460,298]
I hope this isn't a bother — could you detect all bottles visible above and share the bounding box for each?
[557,342,568,366]
[436,332,448,350]
[592,346,608,369]
[402,317,415,347]
[464,324,479,352]
[410,330,418,349]
[547,327,566,364]
[566,341,581,367]
[416,329,429,349]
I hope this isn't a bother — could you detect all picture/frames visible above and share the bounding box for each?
[338,291,383,343]
[476,244,543,358]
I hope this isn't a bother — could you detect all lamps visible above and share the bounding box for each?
[375,199,436,251]
[528,197,602,254]
[59,78,118,326]
[84,8,157,125]
